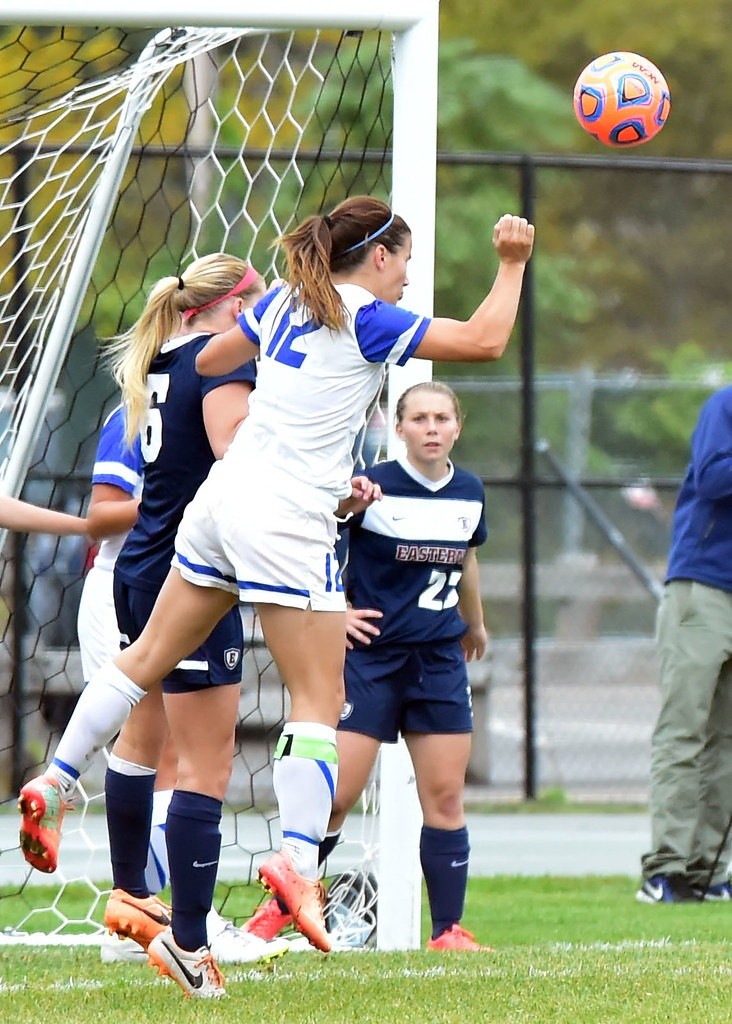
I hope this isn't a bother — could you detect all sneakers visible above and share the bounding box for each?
[636,871,704,903]
[100,929,151,963]
[257,847,331,954]
[690,881,732,902]
[146,921,228,999]
[16,773,66,874]
[239,897,296,942]
[204,904,289,965]
[426,922,494,952]
[102,887,174,953]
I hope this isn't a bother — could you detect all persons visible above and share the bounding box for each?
[76,392,285,965]
[236,382,507,960]
[98,253,384,1003]
[0,495,96,546]
[637,385,732,908]
[17,196,535,956]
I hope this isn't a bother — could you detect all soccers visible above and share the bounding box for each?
[573,50,671,148]
